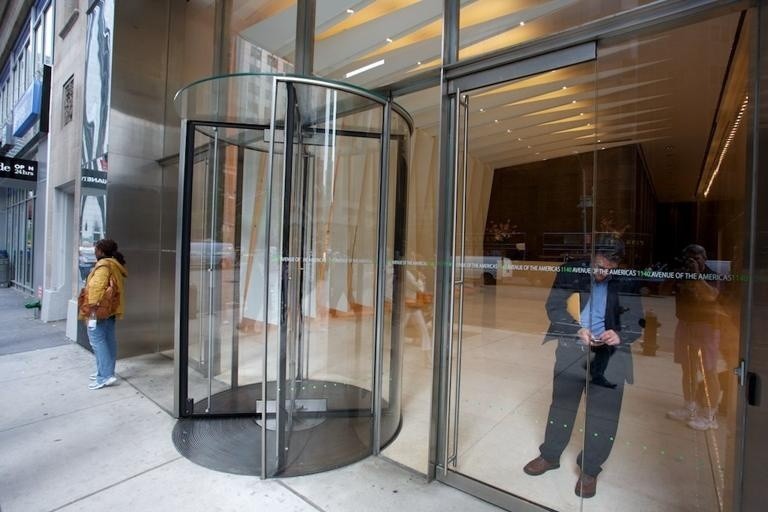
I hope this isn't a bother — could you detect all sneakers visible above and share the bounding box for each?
[688,416,718,430]
[667,408,696,418]
[89,372,116,389]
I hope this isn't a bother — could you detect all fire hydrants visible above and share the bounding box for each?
[637,311,662,359]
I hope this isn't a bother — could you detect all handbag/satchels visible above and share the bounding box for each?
[78,286,120,321]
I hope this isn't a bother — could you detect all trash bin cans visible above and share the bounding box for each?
[0,250,10,288]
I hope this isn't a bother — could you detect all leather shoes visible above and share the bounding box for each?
[523,456,560,474]
[575,473,596,498]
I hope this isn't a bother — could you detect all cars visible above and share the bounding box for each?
[77,245,98,268]
[8,246,30,265]
[616,262,676,297]
[187,238,513,286]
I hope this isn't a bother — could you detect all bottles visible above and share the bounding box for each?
[87,308,97,331]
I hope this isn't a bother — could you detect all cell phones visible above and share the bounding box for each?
[592,337,602,343]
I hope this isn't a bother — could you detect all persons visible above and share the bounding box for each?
[523,236,644,498]
[666,245,722,430]
[405,253,432,352]
[78,239,128,390]
